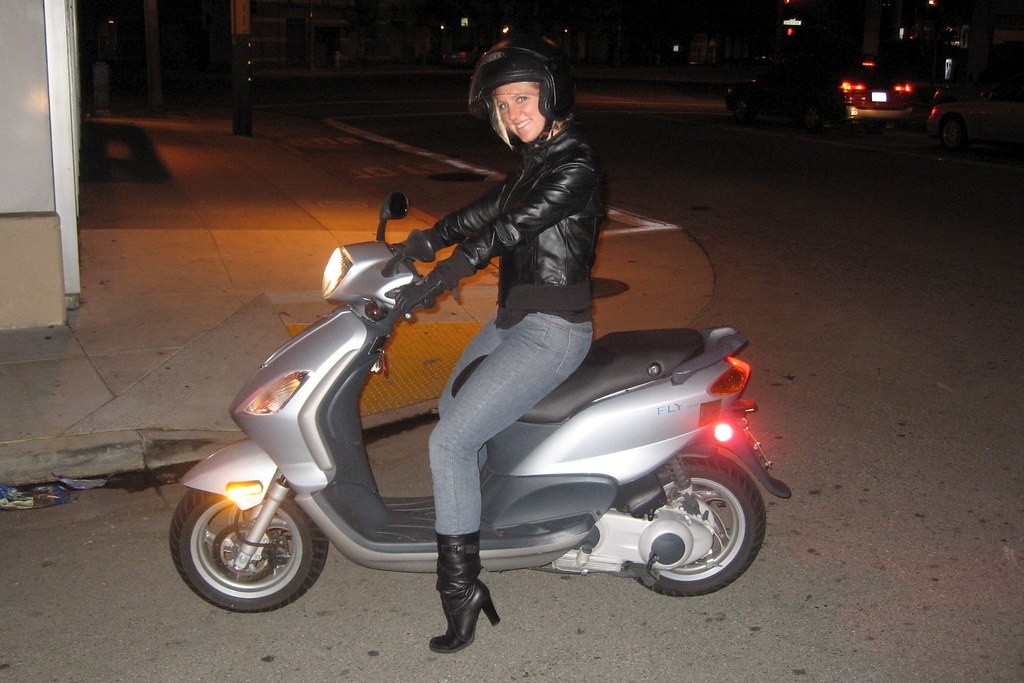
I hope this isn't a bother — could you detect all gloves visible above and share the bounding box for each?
[392,248,475,318]
[388,228,435,267]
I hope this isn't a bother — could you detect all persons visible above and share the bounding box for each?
[388,35,605,654]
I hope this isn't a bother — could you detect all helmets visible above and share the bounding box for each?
[468,34,575,121]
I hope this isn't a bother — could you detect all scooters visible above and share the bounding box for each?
[169,191,793,614]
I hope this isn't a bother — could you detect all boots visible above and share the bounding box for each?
[429,529,500,654]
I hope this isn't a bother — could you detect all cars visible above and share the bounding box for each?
[929,73,1024,153]
[723,42,920,132]
